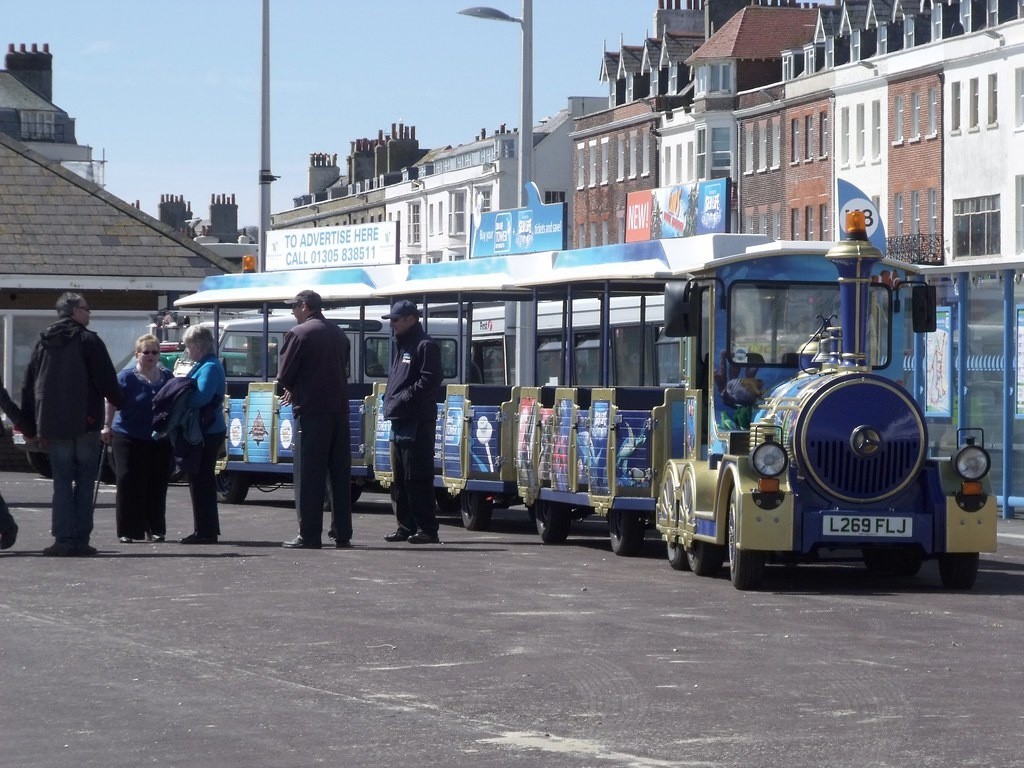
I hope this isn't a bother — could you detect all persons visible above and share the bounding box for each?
[0,380,38,549]
[21,292,128,556]
[101,336,175,542]
[367,347,378,372]
[150,325,227,545]
[381,301,444,543]
[274,291,353,548]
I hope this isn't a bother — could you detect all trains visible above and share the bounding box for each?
[174,211,997,591]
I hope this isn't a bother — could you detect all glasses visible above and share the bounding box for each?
[73,306,89,312]
[291,305,299,311]
[139,350,159,356]
[390,315,408,323]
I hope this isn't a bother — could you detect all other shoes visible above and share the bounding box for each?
[75,543,95,555]
[44,539,76,555]
[1,523,18,549]
[145,530,164,542]
[384,529,408,540]
[408,530,439,544]
[331,537,351,545]
[120,535,132,544]
[281,536,320,547]
[181,528,217,543]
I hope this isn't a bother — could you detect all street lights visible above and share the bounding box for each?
[460,7,535,208]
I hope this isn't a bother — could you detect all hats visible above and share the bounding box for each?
[382,301,416,319]
[284,289,322,310]
